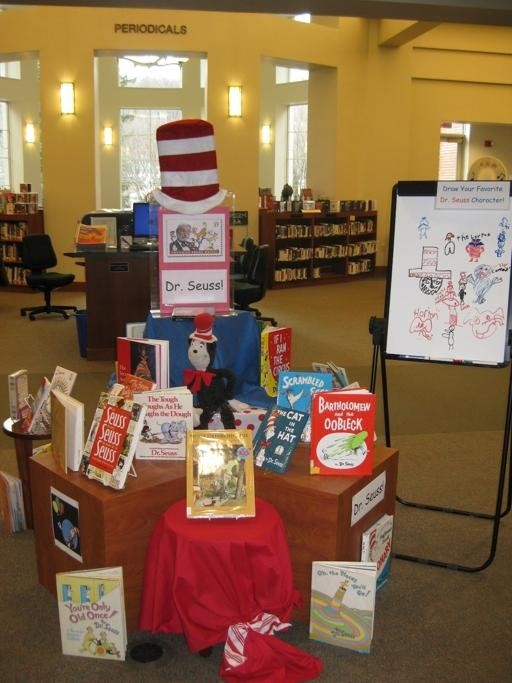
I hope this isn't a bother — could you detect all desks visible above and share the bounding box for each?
[64,247,153,362]
[1,417,51,529]
[161,497,283,658]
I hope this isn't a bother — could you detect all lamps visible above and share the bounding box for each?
[227,85,243,117]
[59,81,75,115]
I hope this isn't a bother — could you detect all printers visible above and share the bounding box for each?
[75,208,134,250]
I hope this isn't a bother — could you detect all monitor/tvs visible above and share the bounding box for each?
[132,203,160,238]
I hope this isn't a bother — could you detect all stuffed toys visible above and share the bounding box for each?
[181,315,236,430]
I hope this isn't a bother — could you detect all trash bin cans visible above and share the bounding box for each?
[73,309,88,357]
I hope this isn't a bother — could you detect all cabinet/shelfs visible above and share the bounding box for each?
[0,209,43,293]
[260,210,377,289]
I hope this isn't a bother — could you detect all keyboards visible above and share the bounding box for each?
[129,243,158,251]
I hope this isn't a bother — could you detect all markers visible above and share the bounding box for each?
[452,359,473,365]
[398,355,430,361]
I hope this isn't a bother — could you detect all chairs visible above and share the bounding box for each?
[18,232,76,319]
[229,236,276,324]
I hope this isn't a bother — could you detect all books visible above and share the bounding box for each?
[78,373,193,491]
[257,185,376,283]
[278,371,332,443]
[0,183,39,287]
[309,387,376,477]
[56,566,131,663]
[156,204,233,317]
[185,430,255,518]
[0,469,28,535]
[30,366,77,435]
[116,337,169,390]
[307,513,397,653]
[260,325,293,397]
[9,368,29,418]
[251,403,309,474]
[49,390,85,474]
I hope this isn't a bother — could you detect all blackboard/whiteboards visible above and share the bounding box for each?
[384,180,512,369]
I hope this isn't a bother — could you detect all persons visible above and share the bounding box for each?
[167,220,203,252]
